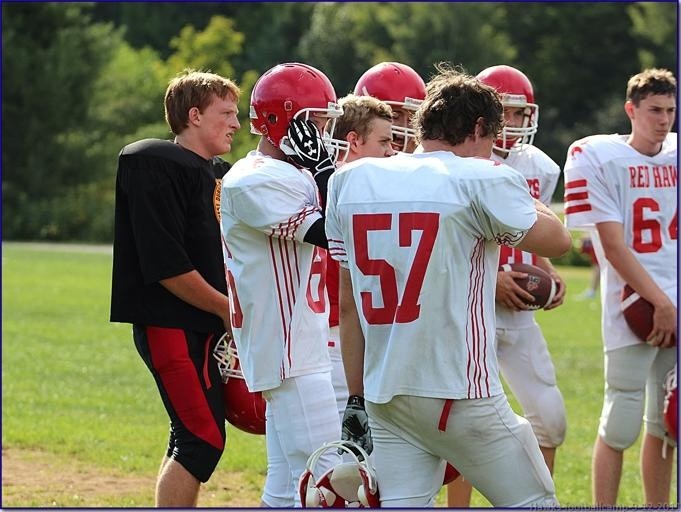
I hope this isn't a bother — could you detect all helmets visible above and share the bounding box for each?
[352,61,429,154]
[298,462,381,509]
[664,365,680,448]
[248,62,341,156]
[473,64,536,150]
[222,336,267,435]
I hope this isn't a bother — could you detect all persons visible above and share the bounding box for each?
[216,61,345,508]
[412,64,569,479]
[110,68,265,508]
[351,62,474,508]
[561,67,679,507]
[324,61,575,509]
[323,91,398,470]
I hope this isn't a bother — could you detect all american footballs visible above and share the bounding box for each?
[620,284,676,350]
[496,263,561,312]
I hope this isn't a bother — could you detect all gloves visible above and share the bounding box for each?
[278,119,340,176]
[337,393,374,462]
